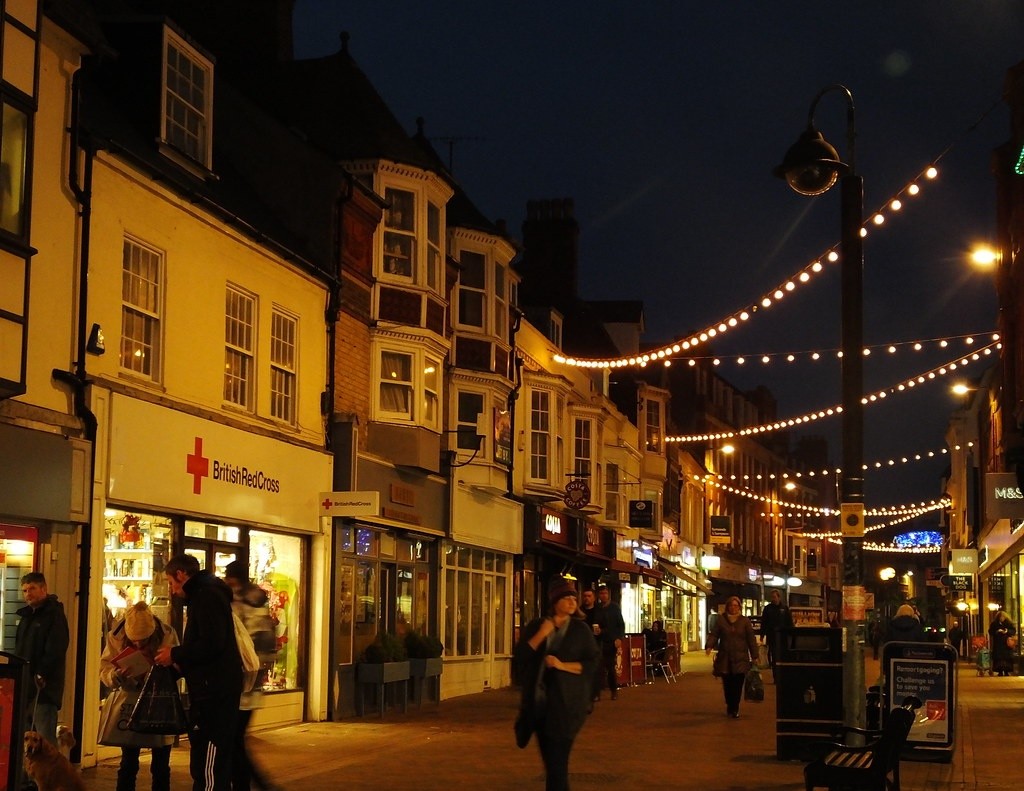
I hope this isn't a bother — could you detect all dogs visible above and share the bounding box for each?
[23,731,90,791]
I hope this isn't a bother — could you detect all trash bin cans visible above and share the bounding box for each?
[774,625,843,759]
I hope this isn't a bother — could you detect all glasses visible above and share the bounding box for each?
[729,605,739,609]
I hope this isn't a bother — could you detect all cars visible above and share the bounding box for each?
[748,616,762,633]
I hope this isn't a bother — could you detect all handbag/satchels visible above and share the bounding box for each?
[1007,637,1016,648]
[125,663,191,735]
[231,611,260,693]
[744,671,764,702]
[96,672,175,748]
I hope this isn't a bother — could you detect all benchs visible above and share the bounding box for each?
[803,694,922,791]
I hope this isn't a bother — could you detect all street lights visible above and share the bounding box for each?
[771,82,867,751]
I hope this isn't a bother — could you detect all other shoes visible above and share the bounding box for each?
[612,693,617,699]
[593,696,600,701]
[734,712,739,717]
[998,673,1003,676]
[1005,674,1009,676]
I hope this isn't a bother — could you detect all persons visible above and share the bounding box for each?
[96,555,277,791]
[579,586,624,701]
[760,589,795,662]
[510,573,601,791]
[868,604,966,660]
[646,621,666,664]
[704,595,758,719]
[988,611,1016,676]
[11,571,69,791]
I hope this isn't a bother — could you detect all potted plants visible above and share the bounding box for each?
[356,629,411,684]
[403,627,445,679]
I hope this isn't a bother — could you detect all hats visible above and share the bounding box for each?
[548,574,578,606]
[124,601,155,641]
